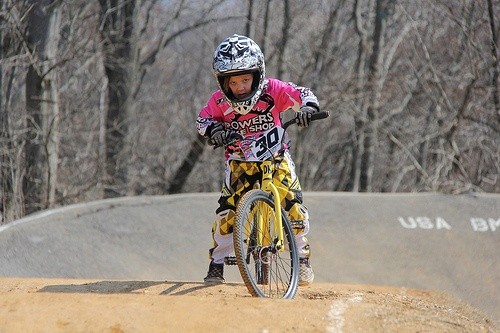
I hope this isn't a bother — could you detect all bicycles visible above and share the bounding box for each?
[207,111,330,300]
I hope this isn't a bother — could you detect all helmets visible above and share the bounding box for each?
[212,34,265,115]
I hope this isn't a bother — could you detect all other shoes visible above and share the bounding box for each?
[298,258,314,285]
[204,261,225,283]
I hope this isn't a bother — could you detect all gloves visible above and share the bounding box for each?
[210,122,227,146]
[295,105,318,128]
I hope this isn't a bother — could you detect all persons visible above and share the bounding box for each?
[196,34,321,288]
